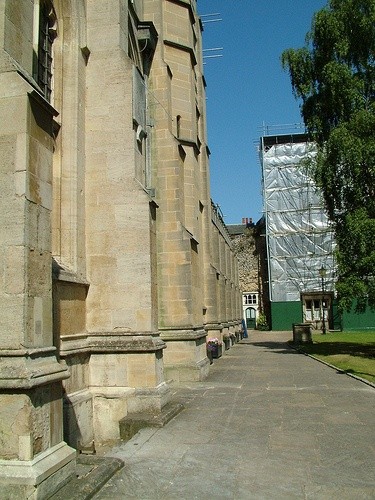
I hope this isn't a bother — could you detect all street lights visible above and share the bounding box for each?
[317,264,328,335]
